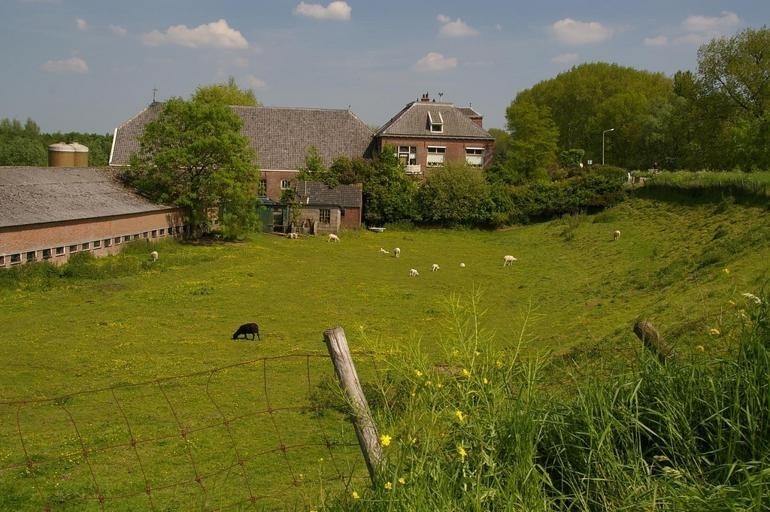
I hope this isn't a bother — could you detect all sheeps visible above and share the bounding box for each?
[410,268,419,277]
[432,263,441,273]
[327,233,341,244]
[503,255,518,268]
[392,247,401,257]
[149,250,160,262]
[458,263,467,268]
[613,229,622,242]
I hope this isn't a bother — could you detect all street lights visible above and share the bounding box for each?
[601,129,615,165]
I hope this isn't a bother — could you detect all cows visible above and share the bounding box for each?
[232,322,261,341]
